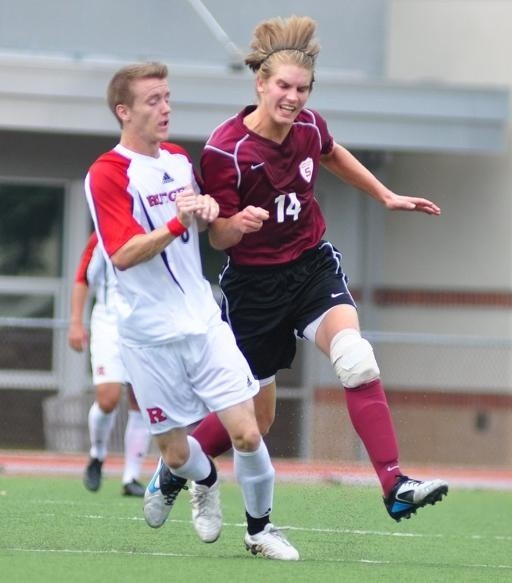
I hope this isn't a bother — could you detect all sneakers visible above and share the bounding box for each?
[191,454,224,543]
[242,521,301,562]
[142,454,189,529]
[81,458,103,492]
[380,473,450,523]
[122,477,147,497]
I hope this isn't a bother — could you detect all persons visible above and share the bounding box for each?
[83,63,304,562]
[141,14,449,528]
[68,225,147,497]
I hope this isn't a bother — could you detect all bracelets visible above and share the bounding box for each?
[167,216,185,240]
